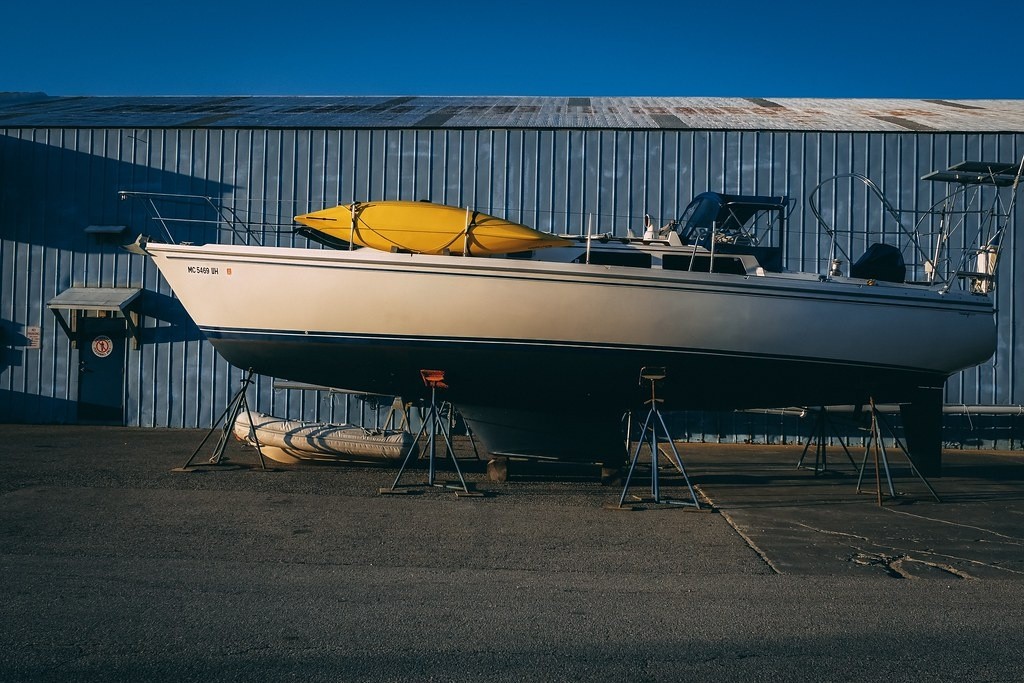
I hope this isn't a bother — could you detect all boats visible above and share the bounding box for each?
[115,159,1024,422]
[293,200,577,257]
[232,408,421,465]
